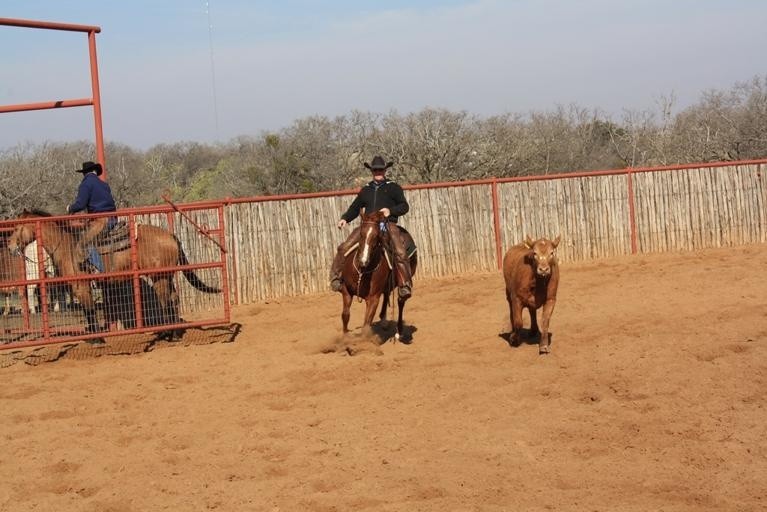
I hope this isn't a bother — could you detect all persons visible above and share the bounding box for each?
[336,155,414,302]
[65,158,118,274]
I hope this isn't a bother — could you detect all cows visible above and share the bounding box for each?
[502,234,561,354]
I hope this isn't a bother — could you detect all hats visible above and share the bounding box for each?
[364,156,393,170]
[76,162,102,176]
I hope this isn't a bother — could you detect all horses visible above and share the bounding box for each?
[7,207,223,342]
[341,207,417,345]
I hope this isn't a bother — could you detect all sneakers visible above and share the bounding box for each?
[398,287,411,302]
[329,272,340,291]
